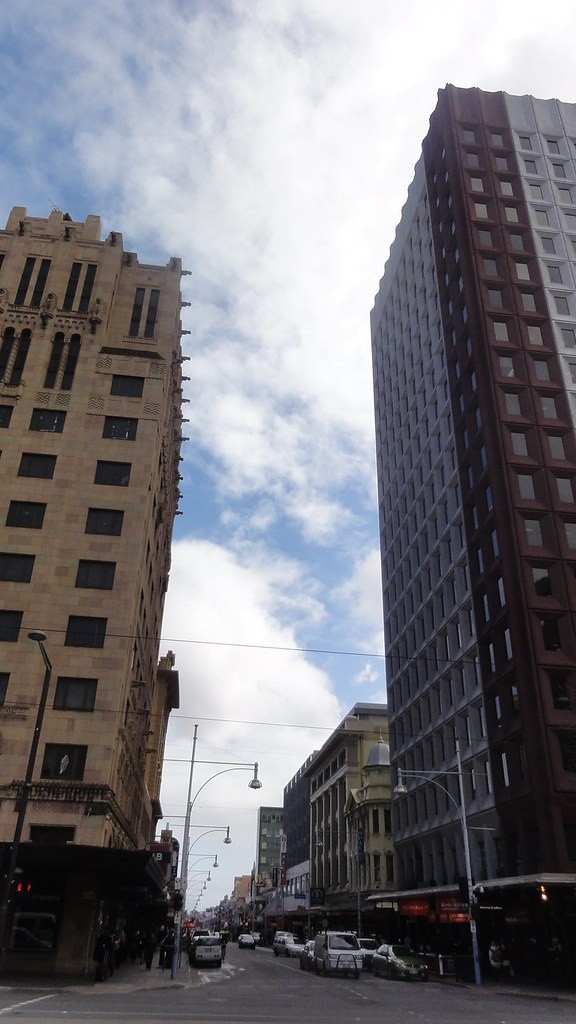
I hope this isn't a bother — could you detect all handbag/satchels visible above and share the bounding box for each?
[93,948,105,963]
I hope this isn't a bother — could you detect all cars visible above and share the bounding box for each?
[187,927,222,969]
[272,931,316,970]
[10,911,57,951]
[238,932,261,950]
[372,943,429,982]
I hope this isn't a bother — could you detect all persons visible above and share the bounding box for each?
[107,926,157,971]
[488,938,511,988]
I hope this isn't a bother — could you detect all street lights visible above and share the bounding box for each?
[0,629,55,954]
[168,723,262,981]
[314,827,378,939]
[393,740,497,987]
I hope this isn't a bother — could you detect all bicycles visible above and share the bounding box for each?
[221,940,227,964]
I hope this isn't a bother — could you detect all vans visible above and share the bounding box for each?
[314,931,363,979]
[356,938,380,969]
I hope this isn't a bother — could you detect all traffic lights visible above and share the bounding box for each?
[11,879,32,894]
